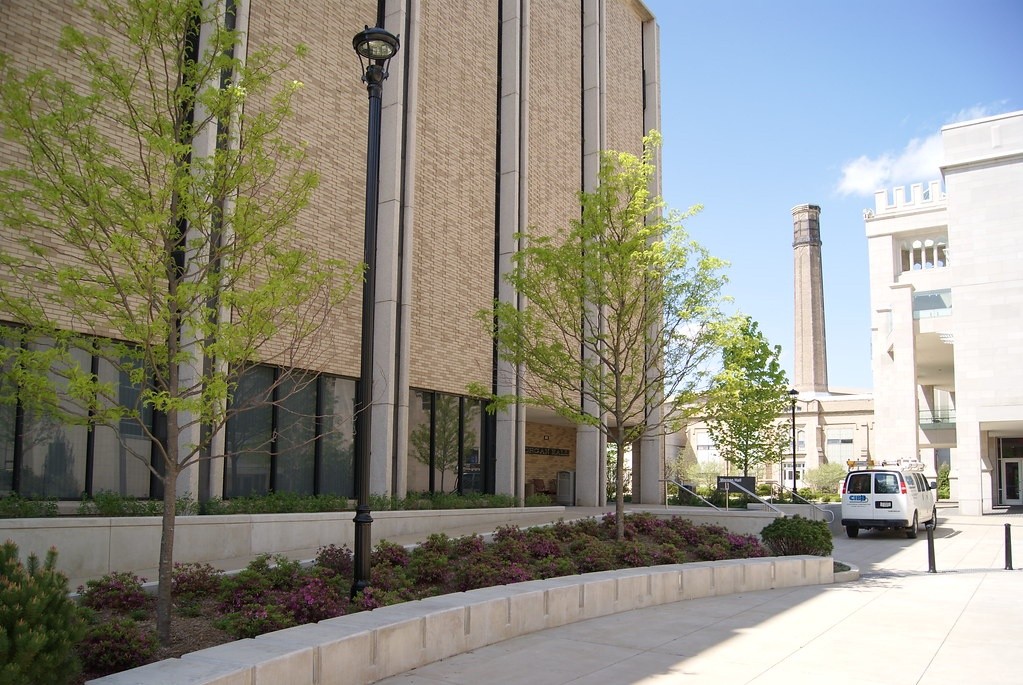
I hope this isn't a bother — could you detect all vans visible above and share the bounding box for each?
[842,469,938,539]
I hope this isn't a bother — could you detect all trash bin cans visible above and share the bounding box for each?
[556,470,575,506]
[679,484,696,505]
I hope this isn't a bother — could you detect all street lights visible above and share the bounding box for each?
[788,387,800,502]
[349,18,402,607]
